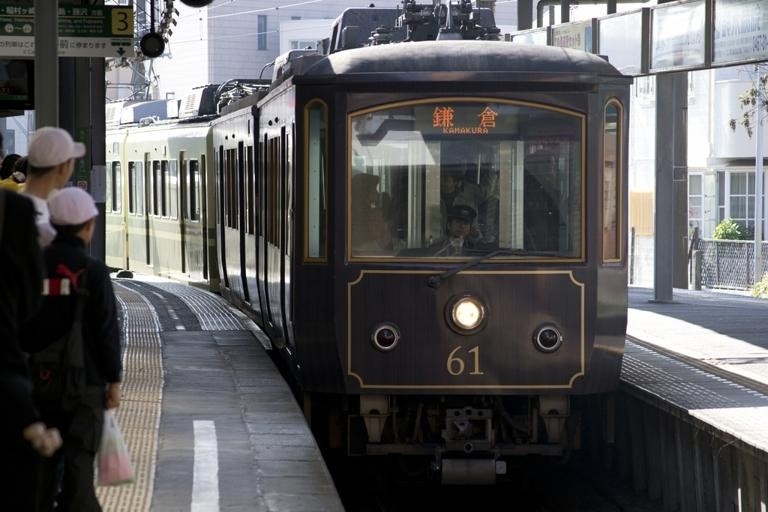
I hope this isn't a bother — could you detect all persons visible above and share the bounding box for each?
[1,127,122,512]
[351,143,498,254]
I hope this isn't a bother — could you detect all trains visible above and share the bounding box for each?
[105,1,635,504]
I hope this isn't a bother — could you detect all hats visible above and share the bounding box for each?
[449,203,476,222]
[46,187,99,225]
[27,126,86,167]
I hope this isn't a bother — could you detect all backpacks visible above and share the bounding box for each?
[30,253,102,398]
